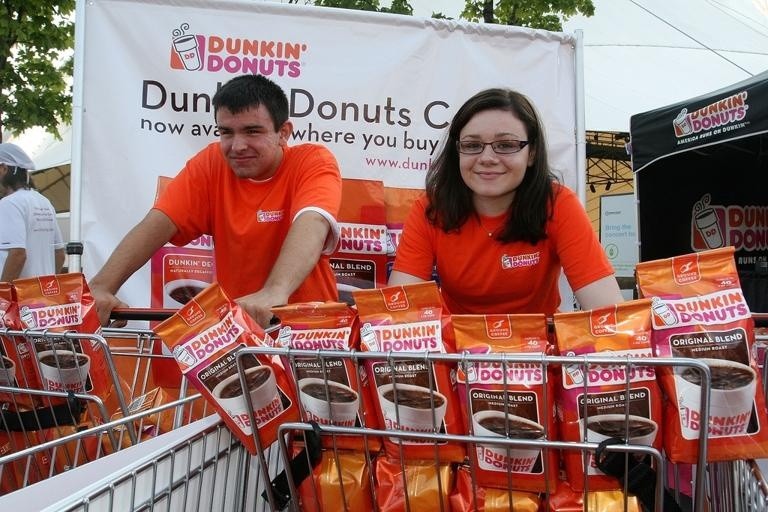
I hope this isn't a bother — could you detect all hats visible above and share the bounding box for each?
[0,144,34,171]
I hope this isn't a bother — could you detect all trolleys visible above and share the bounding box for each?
[1,303,289,511]
[230,273,766,512]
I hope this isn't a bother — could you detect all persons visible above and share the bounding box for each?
[86,74,344,330]
[0,142,67,284]
[388,88,627,313]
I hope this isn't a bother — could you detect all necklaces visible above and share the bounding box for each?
[475,216,507,237]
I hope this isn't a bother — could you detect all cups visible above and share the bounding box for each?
[210,364,284,436]
[579,413,659,478]
[164,278,213,308]
[0,355,16,388]
[298,377,360,427]
[172,35,202,73]
[473,410,548,475]
[37,348,91,397]
[377,382,447,445]
[676,357,757,439]
[674,116,692,135]
[695,207,724,250]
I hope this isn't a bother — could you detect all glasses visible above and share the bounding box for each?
[454,138,531,155]
